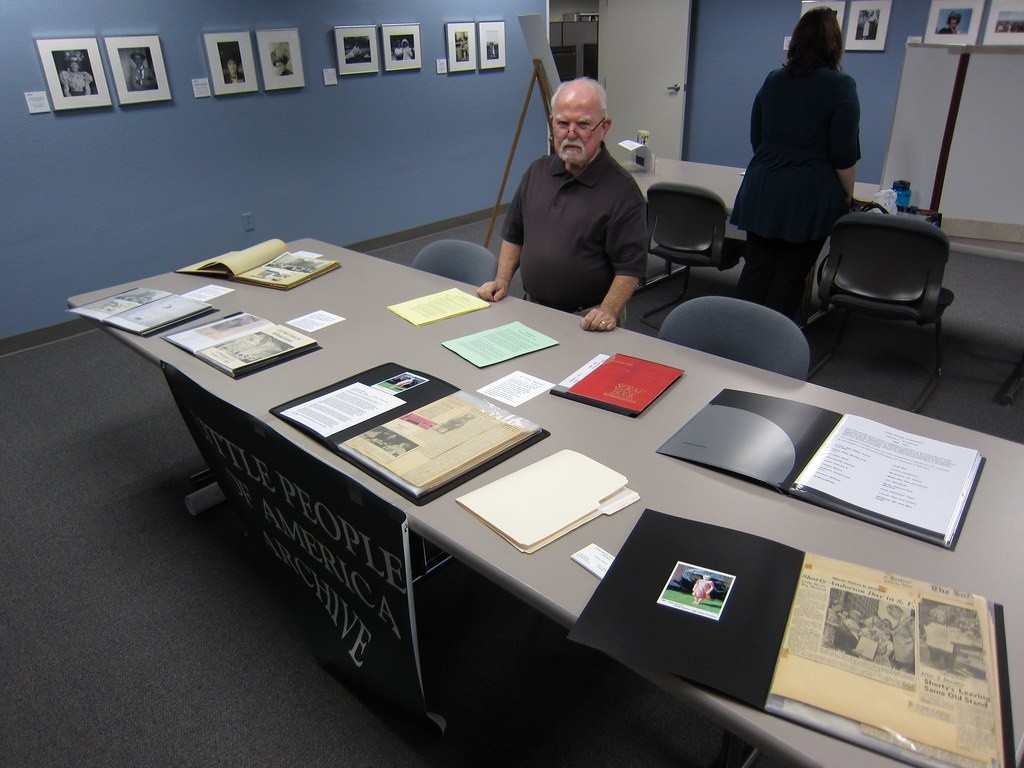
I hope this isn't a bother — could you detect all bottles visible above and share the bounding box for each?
[890,180,912,207]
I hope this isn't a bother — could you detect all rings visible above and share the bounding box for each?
[601,321,607,326]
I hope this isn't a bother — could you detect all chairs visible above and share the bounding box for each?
[637,183,748,330]
[410,240,497,286]
[656,294,809,382]
[799,205,954,413]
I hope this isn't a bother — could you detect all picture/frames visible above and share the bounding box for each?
[801,1,845,33]
[254,27,306,92]
[921,0,985,47]
[477,21,506,71]
[380,22,424,72]
[445,22,478,74]
[201,30,259,97]
[844,1,893,51]
[332,25,380,76]
[35,35,113,112]
[103,34,172,107]
[981,0,1024,51]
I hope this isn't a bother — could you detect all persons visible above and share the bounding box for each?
[391,378,414,388]
[477,77,648,332]
[460,37,468,58]
[488,43,498,59]
[130,51,159,89]
[863,10,878,40]
[59,50,92,96]
[224,56,245,84]
[692,574,714,605]
[937,12,961,35]
[727,9,860,318]
[400,39,412,60]
[270,46,293,76]
[373,434,406,457]
[824,605,915,673]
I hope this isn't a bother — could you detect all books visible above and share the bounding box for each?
[173,238,341,290]
[657,388,987,550]
[161,311,321,380]
[269,361,551,506]
[64,288,220,338]
[565,507,1016,768]
[456,448,639,554]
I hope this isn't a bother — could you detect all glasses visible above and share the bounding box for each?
[551,116,607,140]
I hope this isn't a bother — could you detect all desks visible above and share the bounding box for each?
[66,233,1024,768]
